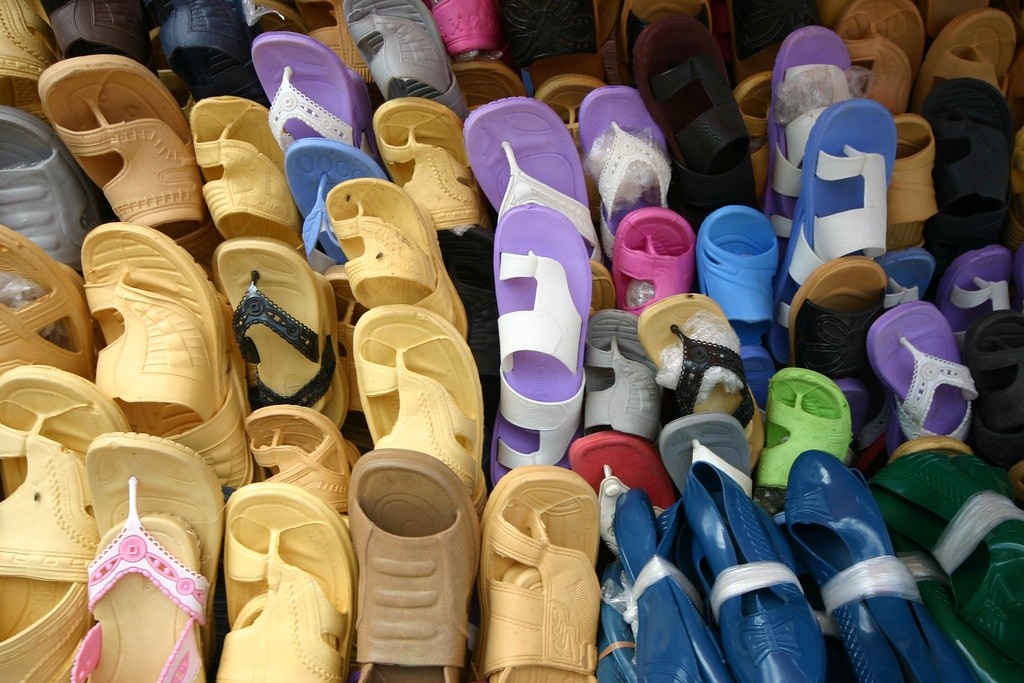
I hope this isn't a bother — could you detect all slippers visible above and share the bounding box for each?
[0,0,1024,683]
